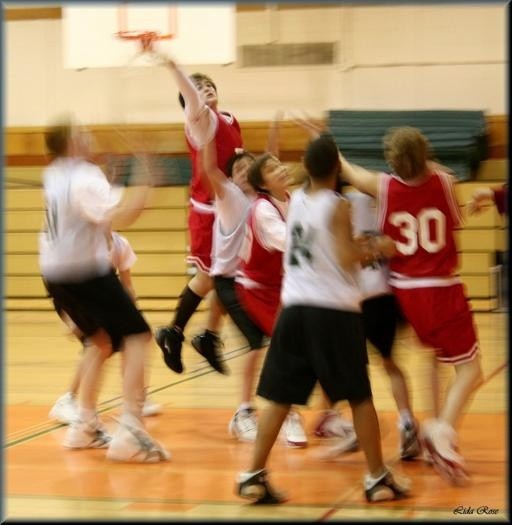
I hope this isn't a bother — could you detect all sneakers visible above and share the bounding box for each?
[122,396,161,415]
[282,412,308,449]
[399,419,420,460]
[329,428,360,457]
[314,410,346,437]
[363,471,410,502]
[420,418,472,488]
[155,325,185,373]
[191,332,232,376]
[232,467,290,504]
[63,418,113,450]
[228,406,258,443]
[106,424,168,463]
[48,392,78,424]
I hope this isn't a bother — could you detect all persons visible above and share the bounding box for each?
[235,137,412,505]
[335,169,423,457]
[465,178,507,217]
[136,35,243,375]
[48,222,163,424]
[37,127,169,465]
[237,154,354,448]
[291,106,484,490]
[197,110,286,443]
